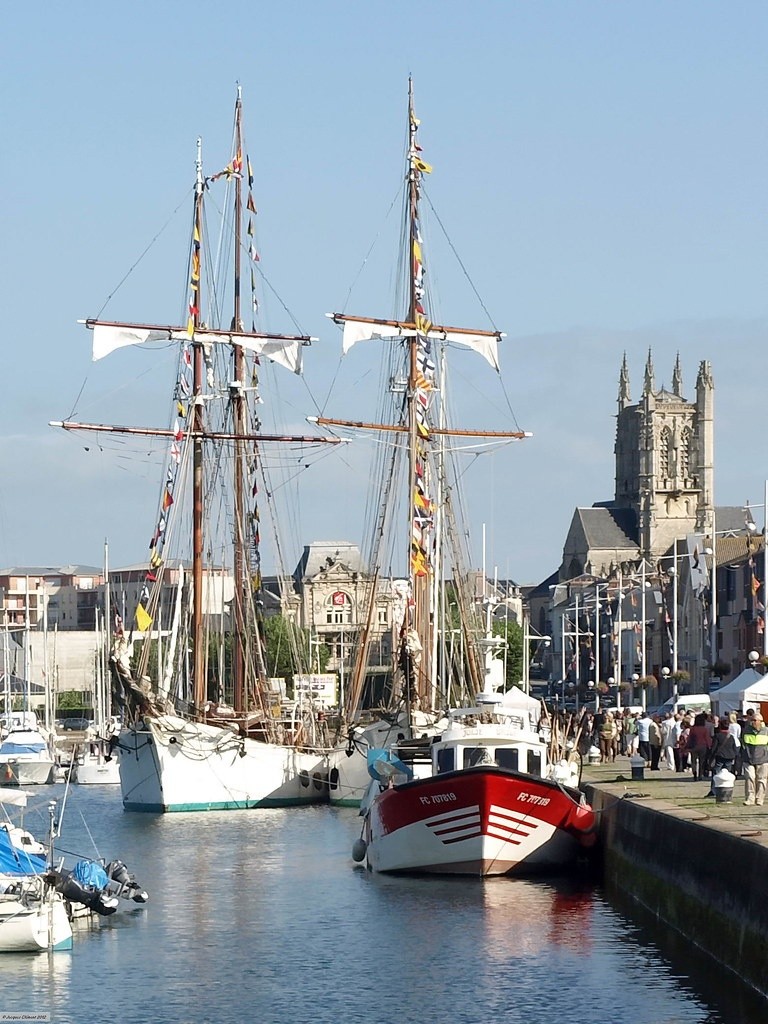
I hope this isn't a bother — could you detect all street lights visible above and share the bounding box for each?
[653,537,716,696]
[629,558,677,718]
[607,571,651,712]
[606,568,655,712]
[565,595,604,714]
[696,513,758,677]
[584,585,627,715]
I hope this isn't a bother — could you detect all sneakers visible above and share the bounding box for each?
[704,791,716,798]
[743,801,755,806]
[756,798,763,805]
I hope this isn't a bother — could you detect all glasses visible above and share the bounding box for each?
[750,719,759,722]
[604,718,609,720]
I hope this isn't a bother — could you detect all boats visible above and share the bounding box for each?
[0,744,149,951]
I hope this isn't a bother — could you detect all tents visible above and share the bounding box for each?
[708,668,768,728]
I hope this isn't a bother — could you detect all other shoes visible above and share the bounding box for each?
[613,758,615,763]
[622,754,626,756]
[600,758,603,762]
[698,777,702,781]
[704,773,708,778]
[644,761,651,768]
[693,777,697,781]
[683,767,692,773]
[628,754,630,757]
[651,767,660,771]
[605,757,608,763]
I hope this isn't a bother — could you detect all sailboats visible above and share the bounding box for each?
[306,71,598,876]
[0,536,121,785]
[46,83,329,812]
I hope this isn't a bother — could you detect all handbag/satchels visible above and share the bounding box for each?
[704,758,716,771]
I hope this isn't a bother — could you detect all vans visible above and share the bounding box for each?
[64,718,89,731]
[0,711,36,732]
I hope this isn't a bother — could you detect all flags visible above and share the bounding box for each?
[134,603,153,632]
[170,442,181,463]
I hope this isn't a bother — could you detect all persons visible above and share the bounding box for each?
[538,705,768,805]
